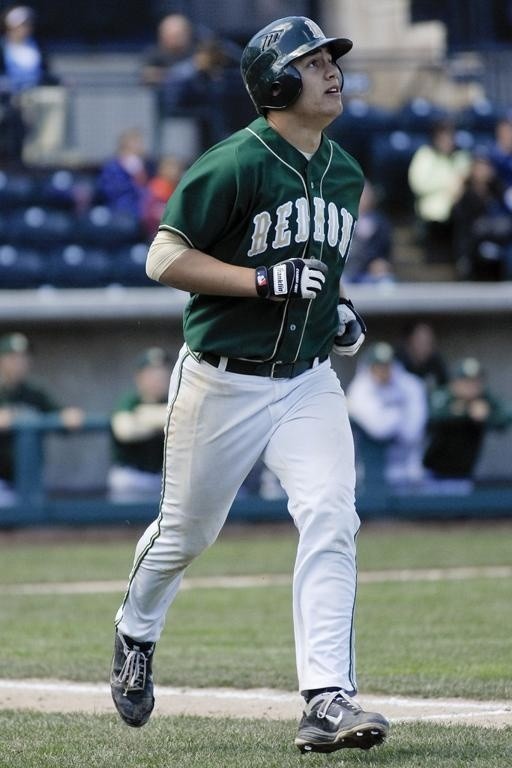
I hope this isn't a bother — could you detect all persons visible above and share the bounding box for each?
[139,13,220,150]
[0,7,60,177]
[97,128,181,244]
[0,334,84,506]
[108,13,391,754]
[348,323,511,494]
[110,347,170,505]
[343,121,512,285]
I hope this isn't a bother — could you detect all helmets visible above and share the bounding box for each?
[240,16,353,118]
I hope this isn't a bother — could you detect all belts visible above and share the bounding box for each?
[202,350,329,378]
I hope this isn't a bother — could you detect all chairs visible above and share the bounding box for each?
[2,94,511,285]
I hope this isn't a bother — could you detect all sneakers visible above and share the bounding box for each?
[294,687,389,753]
[111,628,157,726]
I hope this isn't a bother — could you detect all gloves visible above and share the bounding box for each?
[334,295,368,359]
[255,258,329,304]
